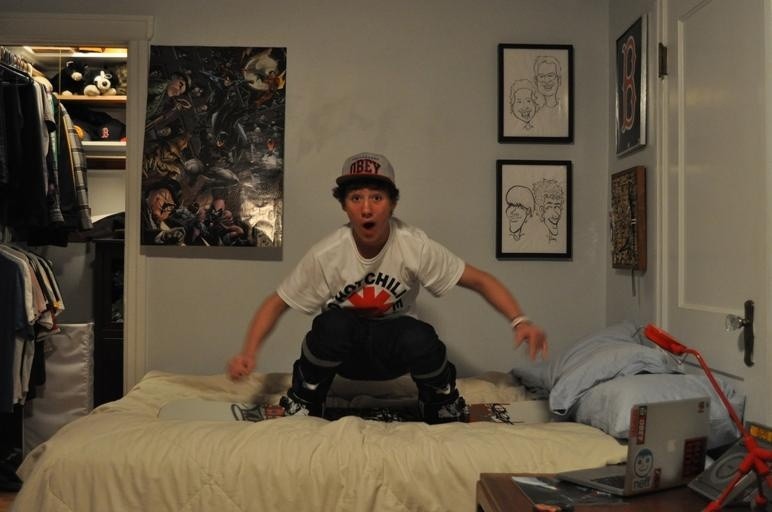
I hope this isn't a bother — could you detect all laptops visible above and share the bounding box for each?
[554,397,711,498]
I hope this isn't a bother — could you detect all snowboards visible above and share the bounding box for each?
[155,397,575,423]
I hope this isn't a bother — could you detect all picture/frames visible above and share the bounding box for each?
[615,13,646,159]
[496,159,574,261]
[497,43,573,144]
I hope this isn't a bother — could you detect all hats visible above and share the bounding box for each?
[335,150,395,187]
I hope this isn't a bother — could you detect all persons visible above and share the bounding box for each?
[227,149,551,424]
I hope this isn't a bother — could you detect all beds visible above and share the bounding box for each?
[9,325,745,512]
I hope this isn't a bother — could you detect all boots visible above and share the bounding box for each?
[409,358,470,423]
[280,335,343,419]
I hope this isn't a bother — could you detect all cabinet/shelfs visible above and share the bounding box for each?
[0,43,127,496]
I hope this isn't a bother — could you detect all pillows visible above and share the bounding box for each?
[509,320,685,416]
[571,365,744,451]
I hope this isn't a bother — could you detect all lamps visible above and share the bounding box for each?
[643,321,772,512]
[722,301,754,368]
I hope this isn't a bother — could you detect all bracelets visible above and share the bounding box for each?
[510,314,529,330]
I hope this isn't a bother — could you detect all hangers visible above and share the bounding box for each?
[0,223,15,246]
[0,45,34,79]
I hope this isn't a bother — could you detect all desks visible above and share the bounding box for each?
[475,463,750,512]
